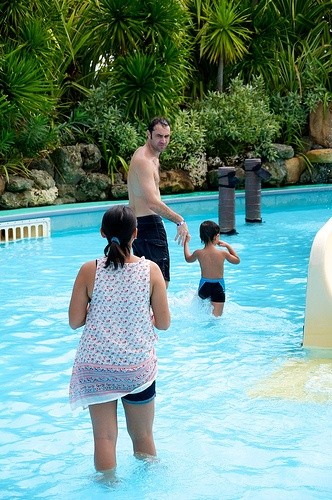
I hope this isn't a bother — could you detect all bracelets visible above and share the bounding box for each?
[177,219,185,227]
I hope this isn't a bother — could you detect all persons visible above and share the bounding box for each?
[184,221,240,317]
[68,205,171,490]
[127,116,187,289]
